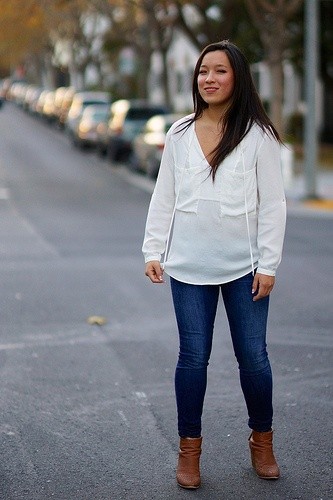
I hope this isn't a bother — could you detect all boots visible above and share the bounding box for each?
[175,436,203,489]
[249,428,280,479]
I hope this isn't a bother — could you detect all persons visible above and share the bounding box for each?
[140,38,287,490]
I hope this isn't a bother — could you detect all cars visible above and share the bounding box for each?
[2,77,191,177]
